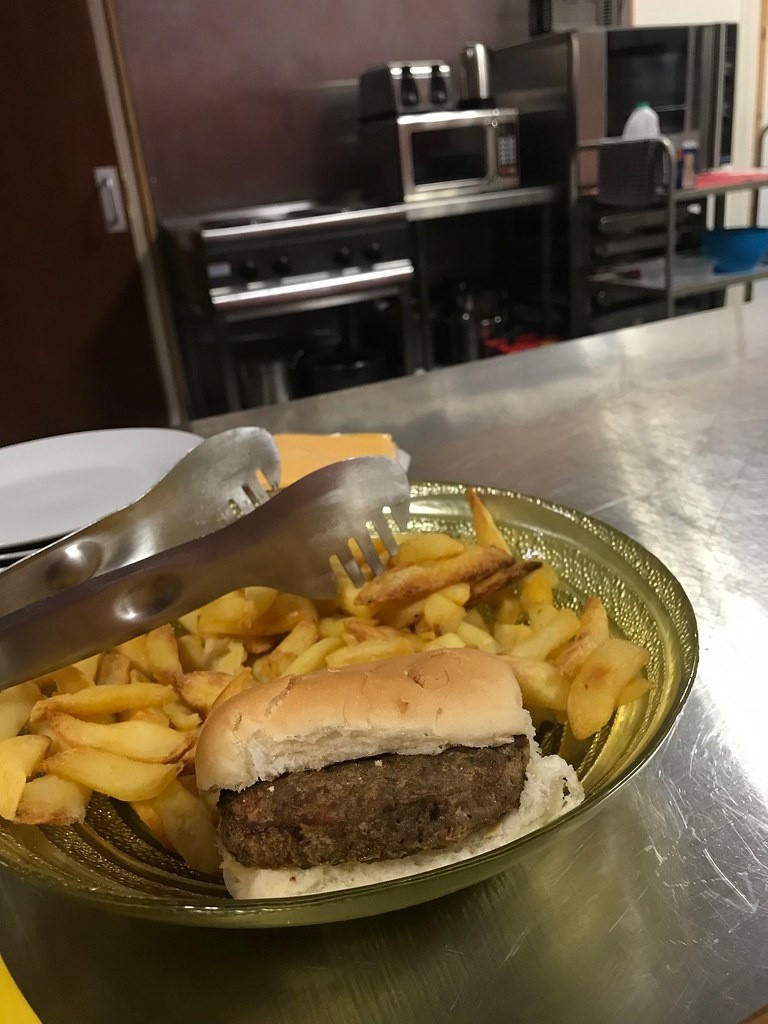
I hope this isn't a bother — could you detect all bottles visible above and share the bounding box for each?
[621,100,662,140]
[675,137,699,190]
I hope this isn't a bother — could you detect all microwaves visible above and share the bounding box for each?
[366,107,526,205]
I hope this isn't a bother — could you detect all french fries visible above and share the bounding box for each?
[0,489,654,871]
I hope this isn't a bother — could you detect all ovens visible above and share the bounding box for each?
[469,16,741,191]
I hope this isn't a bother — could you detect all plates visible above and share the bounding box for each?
[0,427,209,550]
[1,479,698,929]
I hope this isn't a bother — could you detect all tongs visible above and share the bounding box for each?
[1,424,414,693]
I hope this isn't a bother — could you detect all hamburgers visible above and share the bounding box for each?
[194,647,586,901]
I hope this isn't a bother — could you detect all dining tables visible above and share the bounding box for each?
[0,297,768,1024]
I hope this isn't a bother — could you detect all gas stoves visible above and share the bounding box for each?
[160,192,417,325]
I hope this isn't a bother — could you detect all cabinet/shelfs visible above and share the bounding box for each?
[570,126,768,342]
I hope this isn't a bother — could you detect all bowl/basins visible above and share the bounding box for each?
[697,225,768,272]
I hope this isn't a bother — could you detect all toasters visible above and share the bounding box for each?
[355,53,458,119]
